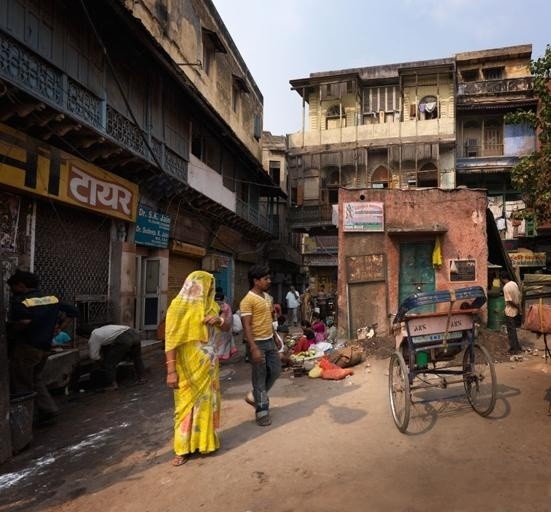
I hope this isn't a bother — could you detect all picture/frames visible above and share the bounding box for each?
[448,257,477,283]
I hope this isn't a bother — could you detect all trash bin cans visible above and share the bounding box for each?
[488,298,505,330]
[10,394,38,454]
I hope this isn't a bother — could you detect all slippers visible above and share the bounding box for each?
[172,455,189,466]
[245,397,272,426]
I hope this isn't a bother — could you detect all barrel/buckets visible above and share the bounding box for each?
[487,295,508,330]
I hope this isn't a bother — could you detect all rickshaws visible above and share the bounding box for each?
[387,286,497,433]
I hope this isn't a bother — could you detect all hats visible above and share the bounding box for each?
[215,287,224,301]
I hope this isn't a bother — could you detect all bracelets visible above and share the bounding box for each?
[216,316,225,327]
[168,370,177,375]
[165,359,177,364]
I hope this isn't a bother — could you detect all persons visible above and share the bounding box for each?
[165,270,233,466]
[233,283,338,369]
[216,286,228,303]
[213,292,239,361]
[75,324,148,392]
[501,270,523,354]
[239,265,285,426]
[7,270,78,427]
